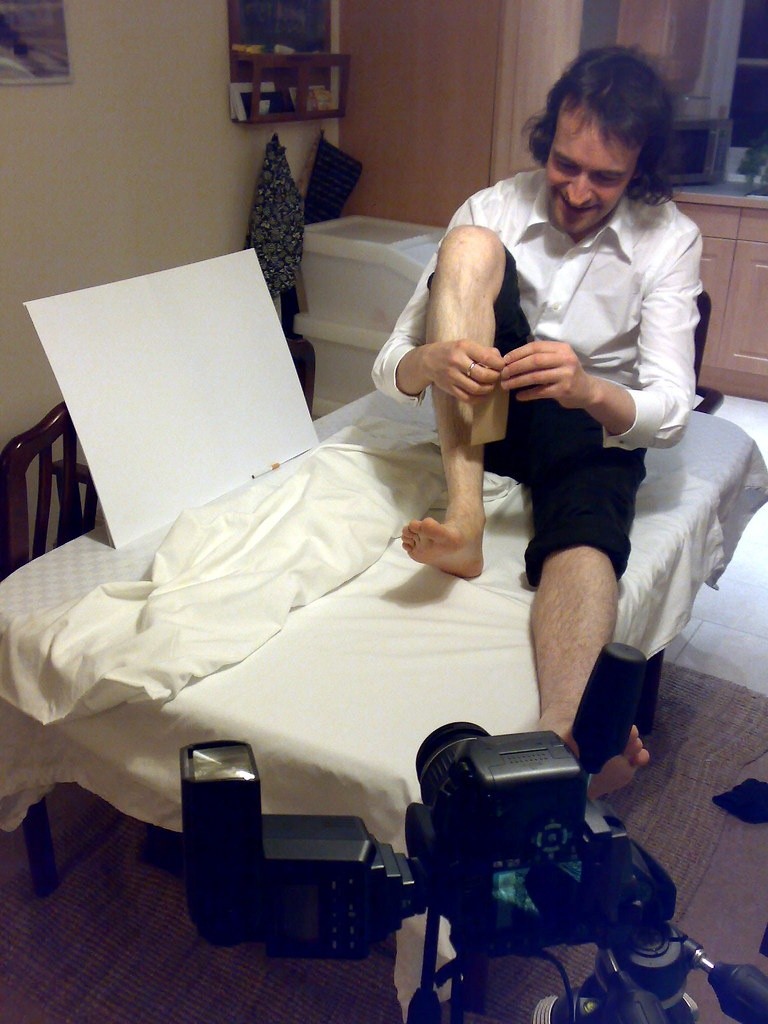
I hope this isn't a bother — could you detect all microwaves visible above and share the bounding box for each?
[657,116,734,184]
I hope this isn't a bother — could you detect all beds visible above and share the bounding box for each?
[0,389,767,919]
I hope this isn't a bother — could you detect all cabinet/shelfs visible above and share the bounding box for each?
[670,202,768,405]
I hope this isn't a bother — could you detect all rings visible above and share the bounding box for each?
[467,361,476,377]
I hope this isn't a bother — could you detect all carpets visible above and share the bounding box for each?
[0,662,768,1024]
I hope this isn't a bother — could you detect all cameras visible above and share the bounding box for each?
[181,721,677,960]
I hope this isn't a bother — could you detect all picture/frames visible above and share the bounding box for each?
[227,1,332,115]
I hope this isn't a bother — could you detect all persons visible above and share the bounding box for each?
[371,42,704,800]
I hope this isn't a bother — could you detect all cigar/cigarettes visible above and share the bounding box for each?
[251,462,279,479]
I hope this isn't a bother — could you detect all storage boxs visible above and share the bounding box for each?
[293,313,393,417]
[293,214,445,332]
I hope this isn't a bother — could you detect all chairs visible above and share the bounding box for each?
[0,401,98,584]
